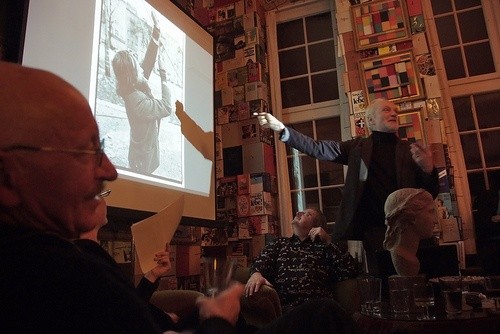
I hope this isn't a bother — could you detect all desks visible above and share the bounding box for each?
[349,296,500,334]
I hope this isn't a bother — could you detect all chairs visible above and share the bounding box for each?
[152,267,282,334]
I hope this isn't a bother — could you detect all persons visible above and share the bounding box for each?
[112,10,175,174]
[238,207,358,328]
[0,63,364,334]
[477,171,500,274]
[253,98,439,295]
[382,188,438,276]
[78,191,178,321]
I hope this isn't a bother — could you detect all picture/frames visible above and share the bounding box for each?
[397,108,429,149]
[357,49,425,107]
[349,0,412,51]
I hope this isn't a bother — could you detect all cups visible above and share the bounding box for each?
[356,277,366,315]
[387,277,409,320]
[438,273,463,319]
[410,279,429,320]
[203,259,234,296]
[360,277,382,317]
[484,277,500,311]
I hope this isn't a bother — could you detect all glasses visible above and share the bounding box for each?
[0,138,105,167]
[99,189,112,198]
